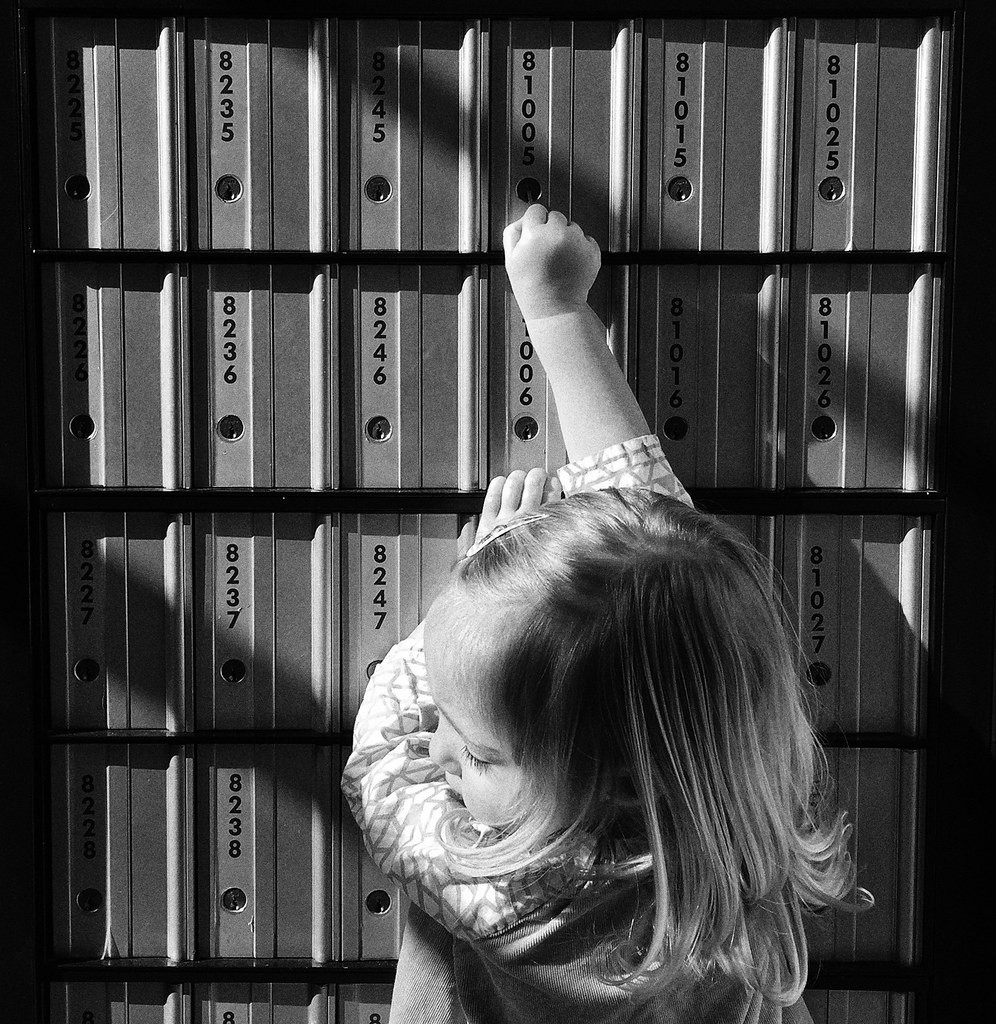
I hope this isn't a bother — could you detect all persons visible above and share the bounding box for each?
[340,204,874,1024]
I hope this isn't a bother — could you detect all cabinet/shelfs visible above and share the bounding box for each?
[0,0,975,1024]
